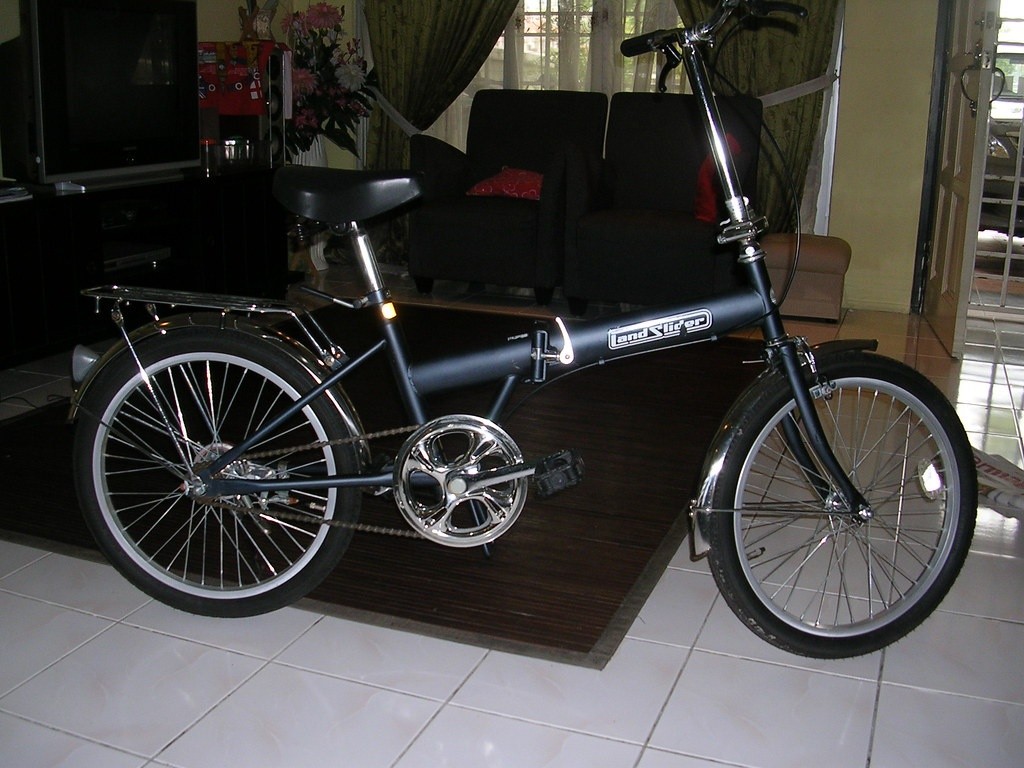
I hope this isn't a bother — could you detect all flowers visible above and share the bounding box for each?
[280,0,380,165]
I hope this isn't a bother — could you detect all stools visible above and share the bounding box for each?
[759,233,852,325]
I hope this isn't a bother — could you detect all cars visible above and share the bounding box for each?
[978,42,1024,240]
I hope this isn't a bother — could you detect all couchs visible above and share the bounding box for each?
[562,92,764,318]
[407,91,608,306]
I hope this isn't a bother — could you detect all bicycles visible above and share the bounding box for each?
[63,0,980,661]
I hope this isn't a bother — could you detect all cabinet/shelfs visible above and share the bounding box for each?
[0,165,289,372]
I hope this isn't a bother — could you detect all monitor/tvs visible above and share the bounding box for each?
[0,0,201,191]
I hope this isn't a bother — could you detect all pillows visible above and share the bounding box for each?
[466,166,544,201]
[693,132,743,224]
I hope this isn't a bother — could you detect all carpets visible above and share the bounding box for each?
[0,297,804,669]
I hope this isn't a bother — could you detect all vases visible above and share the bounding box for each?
[286,132,328,168]
